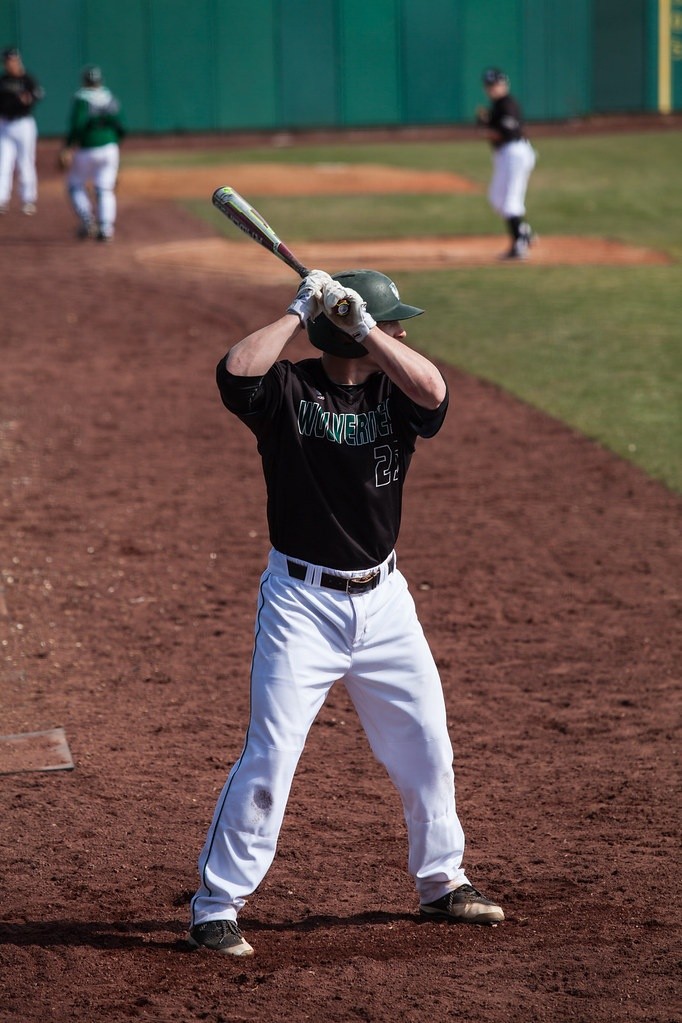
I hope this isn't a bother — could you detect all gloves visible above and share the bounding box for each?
[287,269,333,330]
[315,280,377,343]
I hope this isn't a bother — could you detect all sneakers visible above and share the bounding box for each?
[418,884,506,923]
[189,920,254,958]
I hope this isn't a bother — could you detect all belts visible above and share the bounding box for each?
[287,557,394,596]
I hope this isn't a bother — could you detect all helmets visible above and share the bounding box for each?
[307,269,427,359]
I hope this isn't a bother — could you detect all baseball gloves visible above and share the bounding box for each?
[477,106,489,125]
[58,149,72,171]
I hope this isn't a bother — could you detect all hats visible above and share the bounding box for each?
[483,69,501,84]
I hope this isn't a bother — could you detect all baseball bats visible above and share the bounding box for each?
[210,184,351,317]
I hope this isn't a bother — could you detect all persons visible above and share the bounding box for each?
[58,66,129,241]
[186,268,505,956]
[0,48,48,217]
[476,68,536,260]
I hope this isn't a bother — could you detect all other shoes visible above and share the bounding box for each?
[80,225,98,237]
[508,242,527,257]
[99,233,112,242]
